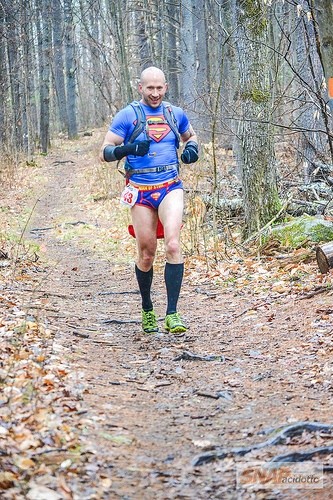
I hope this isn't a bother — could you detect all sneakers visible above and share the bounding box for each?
[141,308,158,333]
[164,312,186,332]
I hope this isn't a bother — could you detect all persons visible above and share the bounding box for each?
[99,66,200,334]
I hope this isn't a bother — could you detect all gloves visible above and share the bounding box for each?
[180,140,198,164]
[102,140,149,162]
